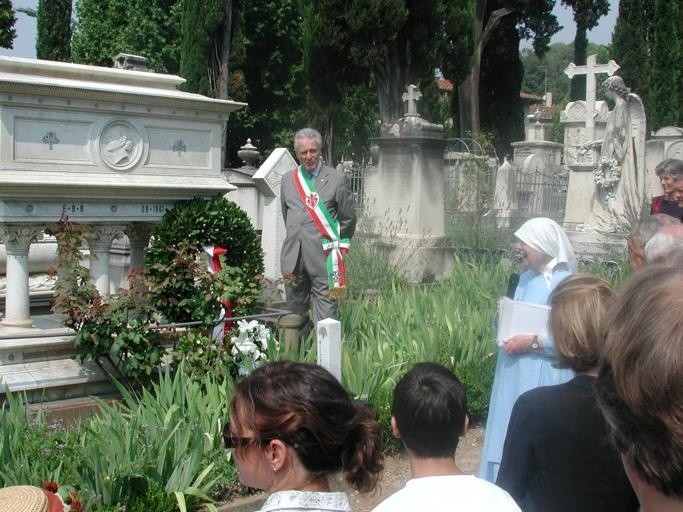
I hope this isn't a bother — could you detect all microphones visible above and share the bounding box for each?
[507,274,519,299]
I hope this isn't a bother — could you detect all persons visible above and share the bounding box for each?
[371,362,521,511]
[479,217,578,483]
[222,360,384,511]
[495,272,641,512]
[280,128,356,347]
[596,247,683,512]
[629,215,683,272]
[651,160,683,223]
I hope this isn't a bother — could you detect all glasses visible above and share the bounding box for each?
[223,422,272,448]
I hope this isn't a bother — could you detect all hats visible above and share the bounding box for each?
[0,485,66,512]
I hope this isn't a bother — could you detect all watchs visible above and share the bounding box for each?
[531,335,540,349]
